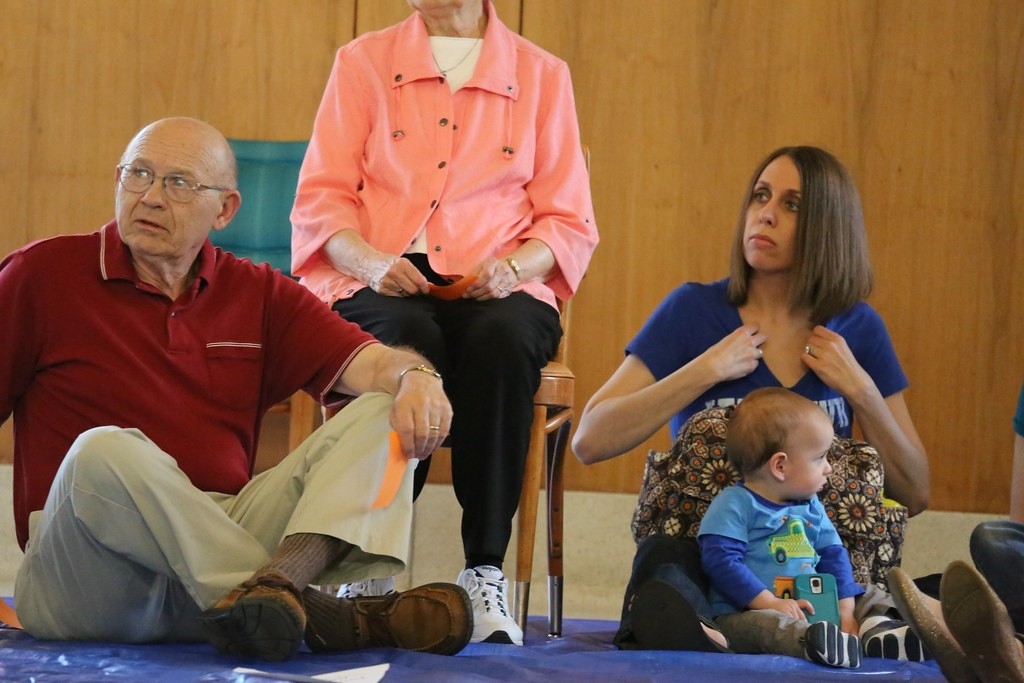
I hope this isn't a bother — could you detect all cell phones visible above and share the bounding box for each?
[793,573,841,629]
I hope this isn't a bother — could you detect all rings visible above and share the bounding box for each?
[758,348,763,357]
[497,286,502,292]
[806,342,815,354]
[395,289,403,294]
[430,426,440,430]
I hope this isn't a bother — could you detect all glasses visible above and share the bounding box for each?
[118,163,227,204]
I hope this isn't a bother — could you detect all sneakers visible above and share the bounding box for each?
[456,565,524,646]
[886,560,1024,683]
[353,581,475,657]
[861,620,930,663]
[336,576,396,597]
[194,583,308,665]
[805,619,865,670]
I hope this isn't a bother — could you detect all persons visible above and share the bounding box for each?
[695,387,935,669]
[885,378,1024,683]
[287,0,601,645]
[0,117,473,659]
[570,147,933,653]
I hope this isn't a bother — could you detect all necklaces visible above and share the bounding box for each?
[430,30,482,75]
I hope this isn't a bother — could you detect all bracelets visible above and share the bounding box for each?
[397,364,442,390]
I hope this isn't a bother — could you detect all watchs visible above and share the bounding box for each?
[506,257,521,282]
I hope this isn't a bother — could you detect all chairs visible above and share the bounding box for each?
[322,146,589,643]
